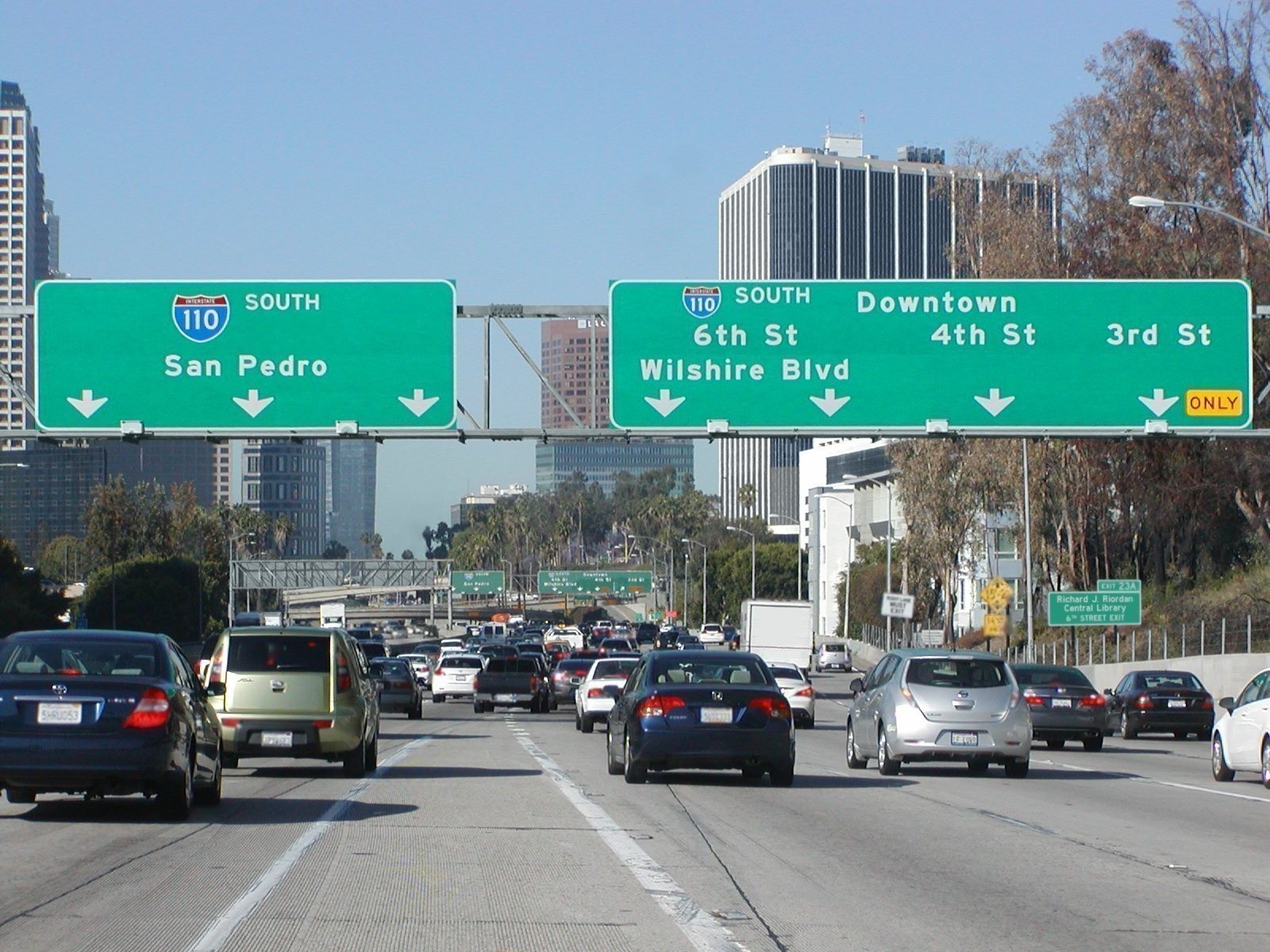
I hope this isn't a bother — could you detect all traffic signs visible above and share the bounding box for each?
[450,571,506,597]
[608,280,1251,436]
[32,279,455,433]
[537,571,652,596]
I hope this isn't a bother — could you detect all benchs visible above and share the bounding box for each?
[11,662,145,676]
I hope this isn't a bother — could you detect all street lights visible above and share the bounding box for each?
[769,514,802,600]
[681,538,706,624]
[813,494,853,641]
[500,559,513,609]
[627,534,674,625]
[227,531,266,628]
[842,475,892,654]
[726,526,755,598]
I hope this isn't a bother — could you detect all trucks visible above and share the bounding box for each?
[318,604,347,631]
[740,601,814,679]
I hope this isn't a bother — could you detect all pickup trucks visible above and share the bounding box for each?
[472,657,550,714]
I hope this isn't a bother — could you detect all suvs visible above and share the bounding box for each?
[205,627,383,779]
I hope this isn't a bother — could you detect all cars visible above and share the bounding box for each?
[39,580,65,597]
[816,643,852,674]
[999,664,1106,752]
[845,648,1031,777]
[0,630,227,823]
[351,615,815,732]
[1209,668,1270,787]
[601,650,796,787]
[1103,670,1215,742]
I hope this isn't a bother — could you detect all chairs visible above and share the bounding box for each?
[658,670,751,684]
[391,670,402,676]
[713,667,741,683]
[447,659,456,666]
